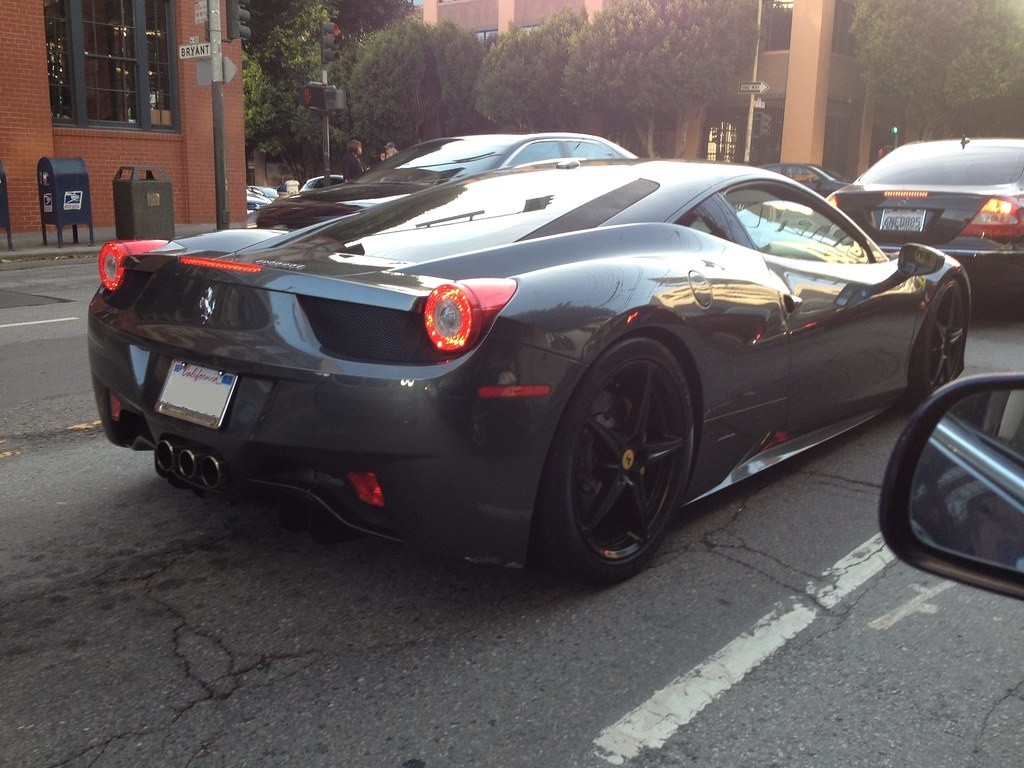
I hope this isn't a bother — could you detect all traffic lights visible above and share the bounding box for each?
[877,146,884,159]
[302,85,328,112]
[891,125,900,135]
[321,19,343,65]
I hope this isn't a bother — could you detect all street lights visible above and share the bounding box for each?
[225,0,253,40]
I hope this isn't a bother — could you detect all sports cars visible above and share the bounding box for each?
[90,152,974,589]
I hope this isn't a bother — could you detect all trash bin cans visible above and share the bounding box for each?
[112,165,175,241]
[38,157,94,247]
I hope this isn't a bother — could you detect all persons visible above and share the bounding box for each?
[341,140,369,184]
[383,142,397,160]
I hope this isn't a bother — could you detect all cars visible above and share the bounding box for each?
[748,162,854,202]
[246,185,280,212]
[825,138,1024,312]
[299,174,345,195]
[254,131,643,233]
